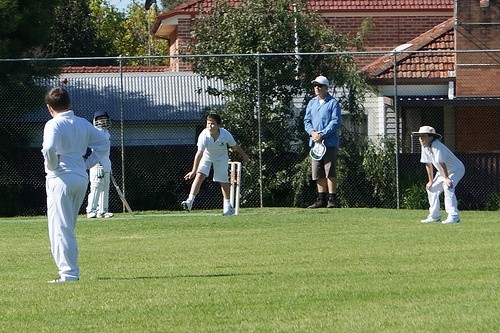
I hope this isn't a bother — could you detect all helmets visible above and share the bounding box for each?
[93,110,111,131]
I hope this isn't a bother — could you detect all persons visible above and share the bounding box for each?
[180,112,253,215]
[40,86,110,282]
[410,125,466,224]
[303,76,341,209]
[85,109,115,218]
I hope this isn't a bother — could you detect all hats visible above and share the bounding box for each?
[311,76,329,86]
[412,125,442,140]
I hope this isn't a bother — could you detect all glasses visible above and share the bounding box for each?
[312,83,327,87]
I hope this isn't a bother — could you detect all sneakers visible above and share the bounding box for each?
[420,214,461,224]
[307,197,339,207]
[46,276,79,282]
[87,210,114,218]
[180,200,192,212]
[222,204,235,216]
